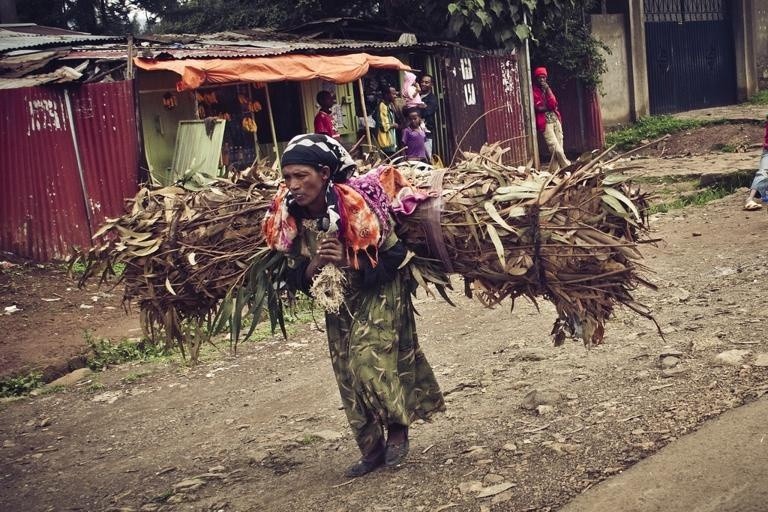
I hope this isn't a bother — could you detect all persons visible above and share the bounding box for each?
[314,90,340,142]
[532,66,572,179]
[360,72,437,165]
[743,115,768,209]
[262,133,445,479]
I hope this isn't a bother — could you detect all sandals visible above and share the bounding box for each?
[742,202,762,210]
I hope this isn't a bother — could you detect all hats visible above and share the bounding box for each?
[533,68,547,77]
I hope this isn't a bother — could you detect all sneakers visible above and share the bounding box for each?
[346,450,384,477]
[385,425,409,465]
